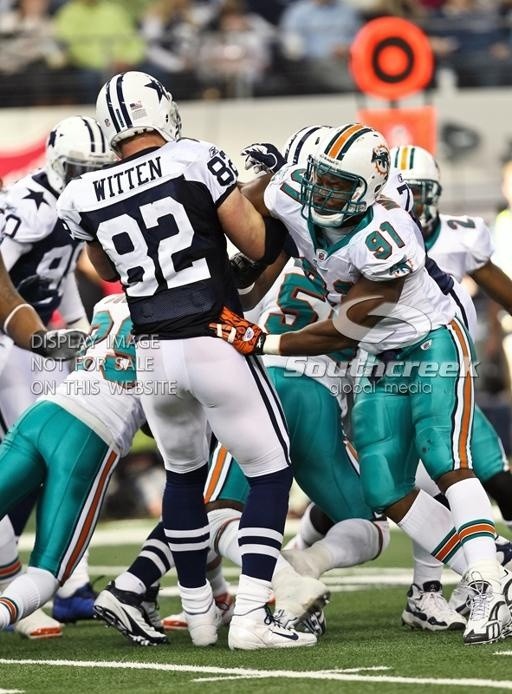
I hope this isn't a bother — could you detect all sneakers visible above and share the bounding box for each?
[14,608,62,639]
[399,534,512,645]
[162,575,331,652]
[92,579,169,646]
[52,575,104,624]
[141,581,165,633]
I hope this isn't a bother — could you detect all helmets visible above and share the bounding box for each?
[45,115,116,195]
[299,123,391,178]
[283,124,323,163]
[95,69,182,157]
[389,144,442,189]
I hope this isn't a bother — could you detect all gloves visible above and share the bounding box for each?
[241,141,286,174]
[207,305,266,355]
[28,329,86,361]
[228,254,270,288]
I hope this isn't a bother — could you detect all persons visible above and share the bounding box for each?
[61,72,315,649]
[0,119,512,644]
[2,2,510,101]
